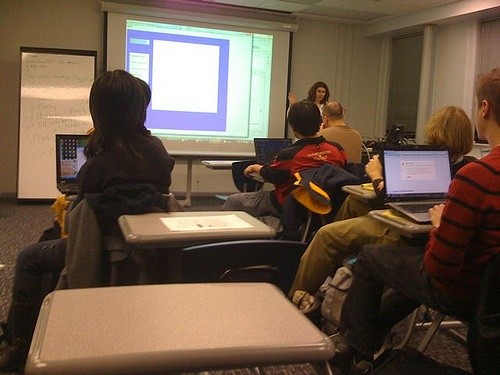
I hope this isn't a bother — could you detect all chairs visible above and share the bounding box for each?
[181,239,308,295]
[232,160,263,201]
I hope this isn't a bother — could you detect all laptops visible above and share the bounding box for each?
[378,125,404,144]
[55,134,90,195]
[380,144,455,224]
[254,138,293,163]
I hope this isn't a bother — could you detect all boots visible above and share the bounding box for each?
[0,337,30,375]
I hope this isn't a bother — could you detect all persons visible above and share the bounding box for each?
[314,102,363,164]
[287,81,329,145]
[218,102,348,219]
[285,106,482,304]
[329,68,500,375]
[0,70,176,375]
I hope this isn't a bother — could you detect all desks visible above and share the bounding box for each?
[368,208,467,346]
[118,211,277,280]
[200,160,248,170]
[24,282,336,375]
[167,149,256,206]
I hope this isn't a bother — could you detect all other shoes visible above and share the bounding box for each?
[350,358,371,375]
[327,334,352,366]
